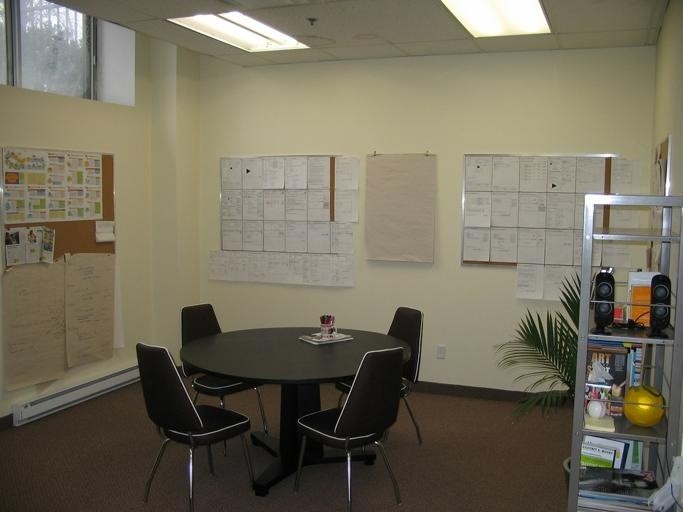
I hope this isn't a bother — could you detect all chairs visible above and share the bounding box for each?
[294,345,405,510]
[335,305,424,452]
[177,303,268,461]
[135,341,256,509]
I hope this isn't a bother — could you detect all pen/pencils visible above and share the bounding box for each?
[319,313,335,325]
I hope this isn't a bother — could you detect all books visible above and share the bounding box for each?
[613,271,661,328]
[576,339,673,512]
[299,331,354,345]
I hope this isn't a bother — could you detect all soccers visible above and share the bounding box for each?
[625,386,664,426]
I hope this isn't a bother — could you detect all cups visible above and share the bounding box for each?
[320,324,337,340]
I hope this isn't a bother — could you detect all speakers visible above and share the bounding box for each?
[645,275,671,339]
[590,273,614,336]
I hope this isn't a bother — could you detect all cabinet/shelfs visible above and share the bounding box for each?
[565,195,682,512]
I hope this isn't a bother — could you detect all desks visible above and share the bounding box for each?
[180,327,413,466]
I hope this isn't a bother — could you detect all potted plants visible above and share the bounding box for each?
[495,269,595,494]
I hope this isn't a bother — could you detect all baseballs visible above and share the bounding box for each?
[587,400,606,419]
